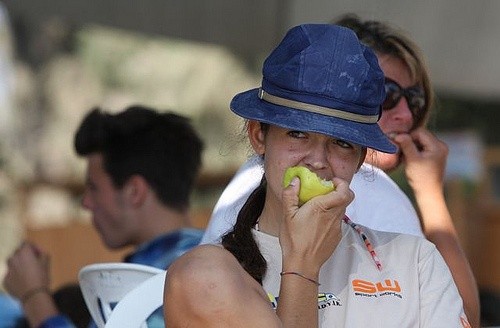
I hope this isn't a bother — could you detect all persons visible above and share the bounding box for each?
[163,24,472,328]
[198,13,480,328]
[2,104,208,328]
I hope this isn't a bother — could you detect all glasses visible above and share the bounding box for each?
[382,77,427,118]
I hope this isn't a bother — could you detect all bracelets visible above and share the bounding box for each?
[22,286,49,306]
[280,272,321,286]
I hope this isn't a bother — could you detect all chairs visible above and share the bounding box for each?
[79,264,167,328]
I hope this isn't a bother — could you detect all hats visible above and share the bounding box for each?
[230,24,398,153]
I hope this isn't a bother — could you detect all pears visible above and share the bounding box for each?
[284,167,336,207]
